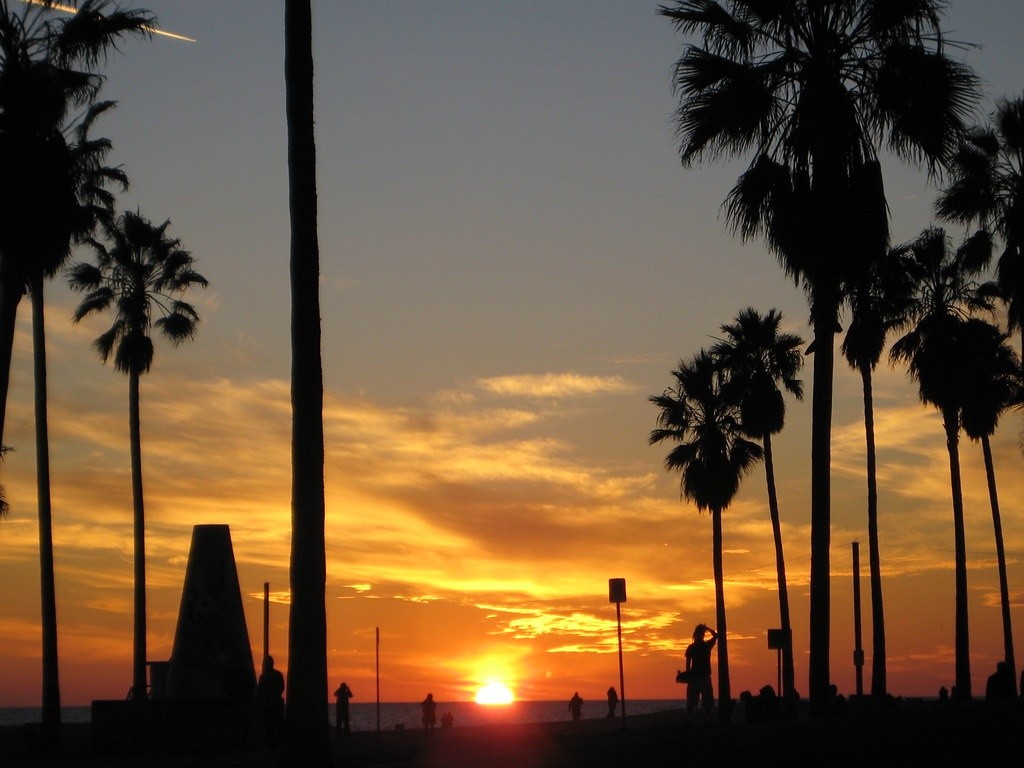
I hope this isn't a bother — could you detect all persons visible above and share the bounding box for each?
[255,655,284,748]
[332,683,353,736]
[685,624,719,710]
[568,693,583,722]
[733,661,1024,722]
[420,694,452,729]
[607,687,619,718]
